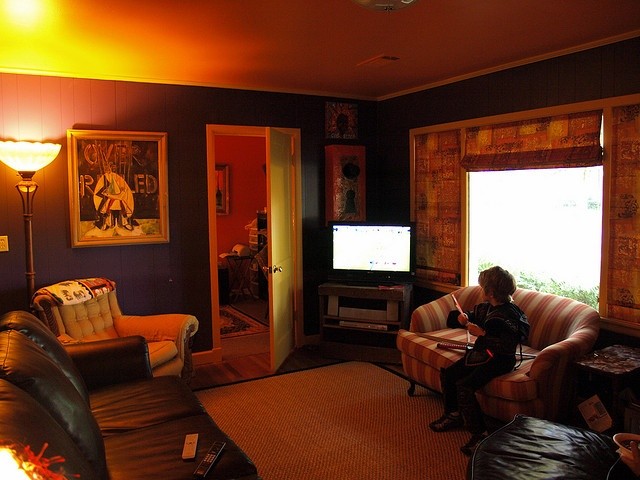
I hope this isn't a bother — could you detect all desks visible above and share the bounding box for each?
[577,344,640,437]
[224,255,254,305]
[316,282,413,365]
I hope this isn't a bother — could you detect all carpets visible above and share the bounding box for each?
[192,360,490,480]
[219,305,269,338]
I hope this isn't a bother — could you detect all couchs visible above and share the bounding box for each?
[466,413,640,480]
[0,310,262,479]
[30,276,199,377]
[396,286,599,424]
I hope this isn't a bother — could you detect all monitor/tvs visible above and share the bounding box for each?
[325,221,416,286]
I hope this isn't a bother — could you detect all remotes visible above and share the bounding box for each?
[193,440,227,478]
[181,430,198,460]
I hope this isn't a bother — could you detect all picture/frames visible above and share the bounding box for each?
[67,129,170,249]
[214,163,230,215]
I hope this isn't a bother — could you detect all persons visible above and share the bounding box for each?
[619,441,640,479]
[429,266,530,457]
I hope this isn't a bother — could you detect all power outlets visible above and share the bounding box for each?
[0,236,9,252]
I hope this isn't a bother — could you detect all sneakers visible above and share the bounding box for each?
[461,434,490,456]
[429,413,465,432]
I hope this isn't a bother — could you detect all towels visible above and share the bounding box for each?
[30,276,115,309]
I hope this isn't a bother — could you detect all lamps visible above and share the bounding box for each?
[0,141,62,314]
